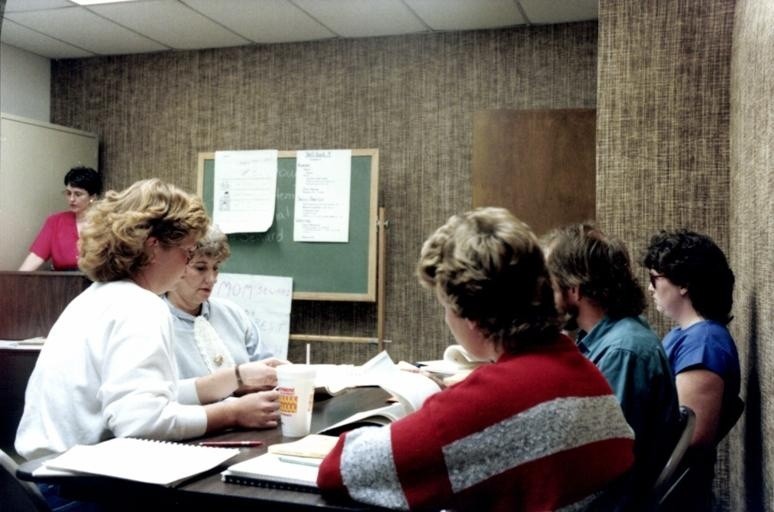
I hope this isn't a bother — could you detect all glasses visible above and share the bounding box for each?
[650,274,665,287]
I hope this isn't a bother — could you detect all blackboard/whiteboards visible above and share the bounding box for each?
[196,148,380,302]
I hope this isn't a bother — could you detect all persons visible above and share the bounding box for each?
[17,166,103,271]
[13,177,294,512]
[541,218,681,483]
[317,206,636,512]
[161,221,278,380]
[639,227,742,462]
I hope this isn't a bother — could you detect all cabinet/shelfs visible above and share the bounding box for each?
[0,340,42,450]
[0,266,92,343]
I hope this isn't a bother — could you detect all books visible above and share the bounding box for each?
[31,343,491,494]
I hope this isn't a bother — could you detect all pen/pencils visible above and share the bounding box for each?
[199,441,264,447]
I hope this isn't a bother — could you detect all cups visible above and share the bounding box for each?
[275,364,317,438]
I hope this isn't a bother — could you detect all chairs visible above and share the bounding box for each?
[711,396,743,440]
[548,464,637,512]
[650,403,699,512]
[1,448,53,512]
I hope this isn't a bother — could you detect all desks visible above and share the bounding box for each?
[14,377,385,512]
